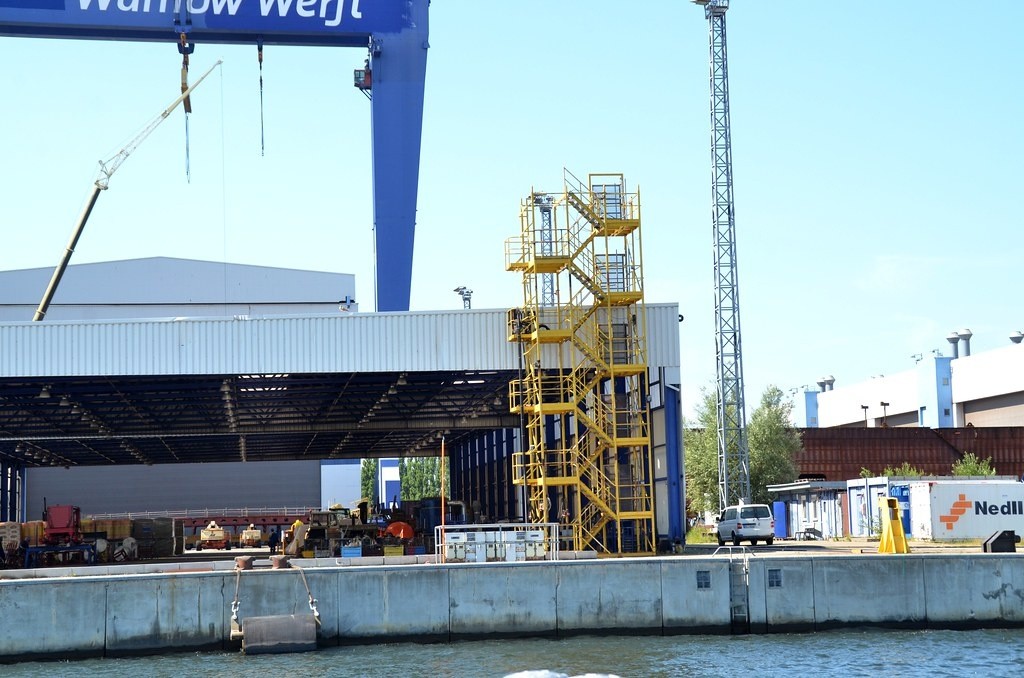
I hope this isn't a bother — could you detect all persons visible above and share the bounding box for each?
[267,528,280,555]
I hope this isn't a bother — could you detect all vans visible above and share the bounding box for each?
[716,504,774,546]
